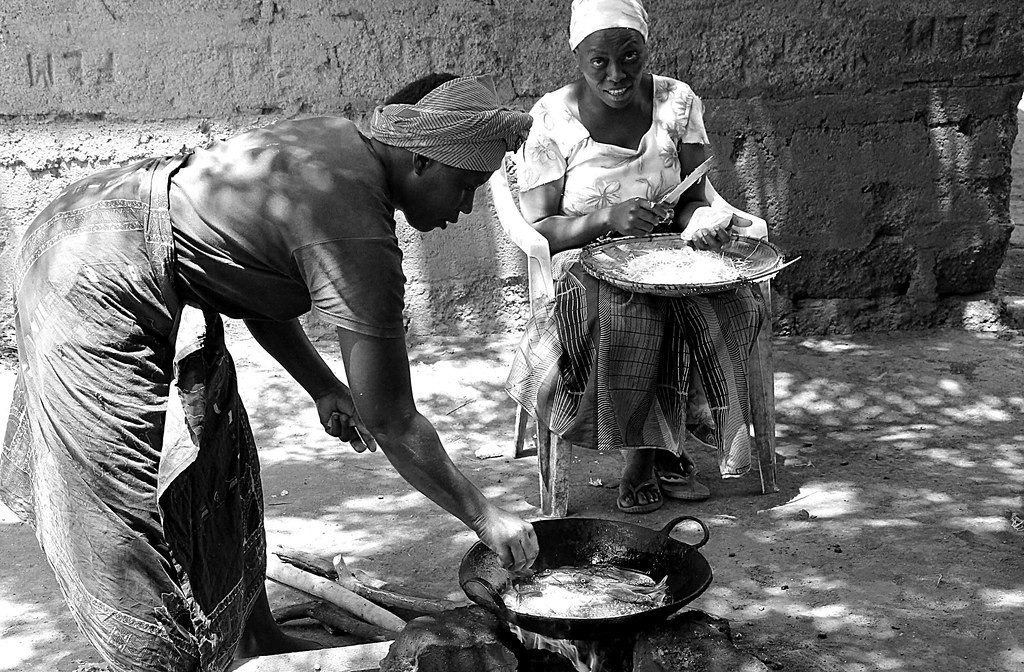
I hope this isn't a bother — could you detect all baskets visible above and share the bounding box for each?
[579,231,801,297]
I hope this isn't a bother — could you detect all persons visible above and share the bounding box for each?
[504,0,768,514]
[0,76,541,672]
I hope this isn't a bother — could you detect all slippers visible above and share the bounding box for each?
[617,474,663,513]
[656,466,711,500]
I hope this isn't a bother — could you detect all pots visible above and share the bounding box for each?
[458,516,713,642]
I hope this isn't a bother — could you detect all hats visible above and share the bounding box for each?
[568,0,649,51]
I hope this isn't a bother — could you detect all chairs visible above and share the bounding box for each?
[485,146,779,517]
